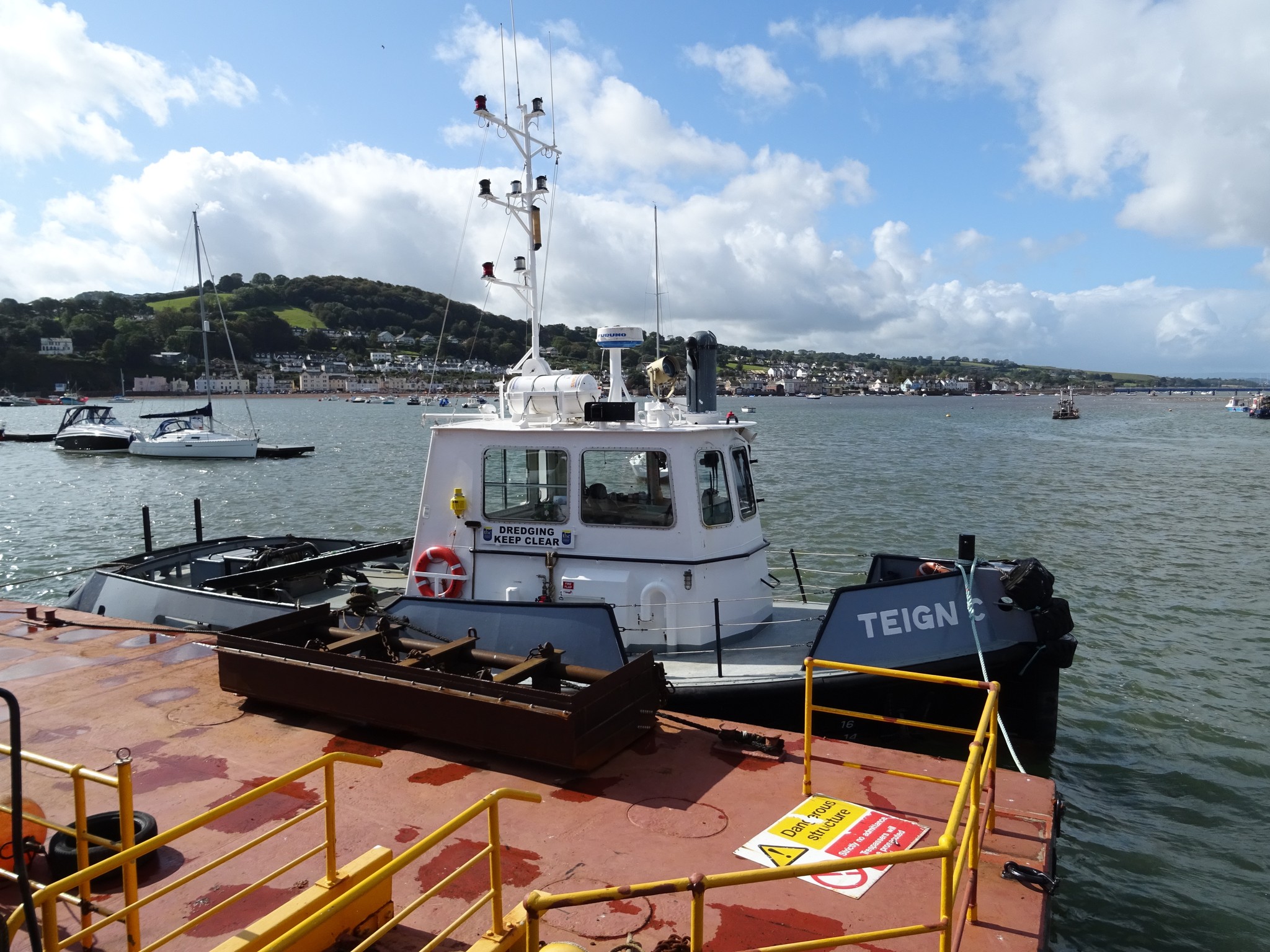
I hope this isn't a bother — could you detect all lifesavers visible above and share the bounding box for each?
[916,562,950,577]
[48,811,159,887]
[415,546,463,598]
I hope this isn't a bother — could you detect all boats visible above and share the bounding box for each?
[1015,392,1026,396]
[322,392,499,409]
[832,394,843,397]
[741,405,756,413]
[53,2,1084,767]
[51,404,143,453]
[805,394,827,399]
[942,393,953,397]
[0,381,90,407]
[1052,387,1081,420]
[785,393,789,397]
[628,451,669,480]
[1224,391,1270,419]
[796,393,806,397]
[716,394,776,398]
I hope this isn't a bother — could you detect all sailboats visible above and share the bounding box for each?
[106,369,134,403]
[127,200,262,461]
